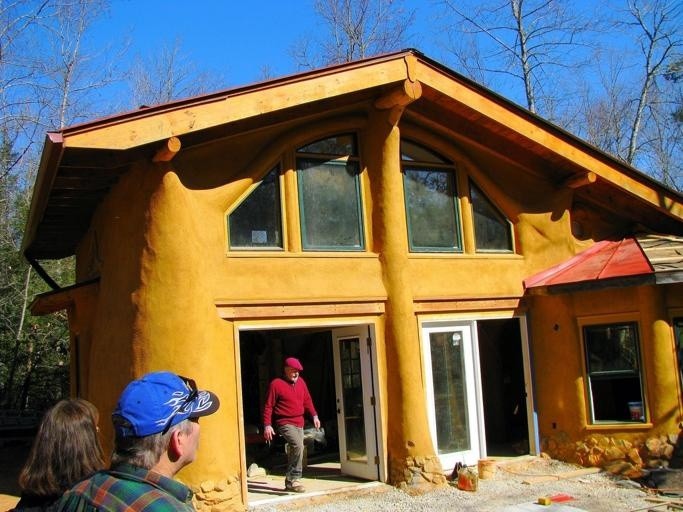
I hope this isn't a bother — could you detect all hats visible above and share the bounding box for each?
[111,370,221,439]
[284,357,303,371]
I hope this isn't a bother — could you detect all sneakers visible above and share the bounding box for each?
[284,481,305,493]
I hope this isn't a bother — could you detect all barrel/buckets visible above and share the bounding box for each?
[478,459,497,479]
[629,402,643,420]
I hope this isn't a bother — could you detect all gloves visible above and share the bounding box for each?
[312,415,320,431]
[264,426,275,443]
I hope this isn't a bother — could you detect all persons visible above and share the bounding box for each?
[5,396,111,511]
[56,371,220,511]
[260,355,323,495]
